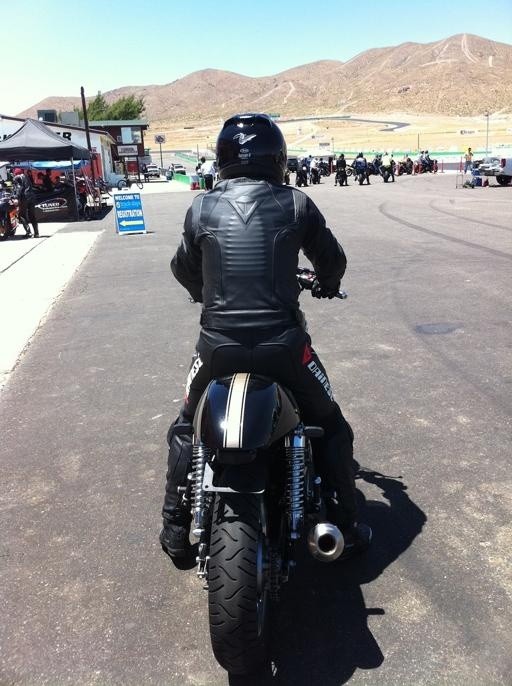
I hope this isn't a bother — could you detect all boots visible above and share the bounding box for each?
[159,417,194,558]
[304,401,367,517]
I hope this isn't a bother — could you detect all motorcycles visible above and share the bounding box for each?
[0,191,17,241]
[165,269,368,680]
[293,160,438,186]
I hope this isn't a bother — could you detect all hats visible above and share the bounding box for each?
[13,168,23,176]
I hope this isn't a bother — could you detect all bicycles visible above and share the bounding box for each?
[116,175,144,190]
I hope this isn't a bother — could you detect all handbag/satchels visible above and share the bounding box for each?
[22,186,35,205]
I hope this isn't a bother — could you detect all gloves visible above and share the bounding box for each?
[311,278,340,299]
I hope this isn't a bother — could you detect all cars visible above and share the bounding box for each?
[143,164,160,177]
[287,157,298,172]
[474,156,512,189]
[170,164,186,176]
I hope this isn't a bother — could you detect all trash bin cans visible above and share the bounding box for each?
[166,171,171,180]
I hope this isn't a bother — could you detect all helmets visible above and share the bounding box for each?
[217,113,287,186]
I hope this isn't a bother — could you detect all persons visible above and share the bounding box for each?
[464,148,473,174]
[142,163,149,183]
[159,113,373,562]
[169,163,174,177]
[8,167,54,238]
[196,150,433,191]
